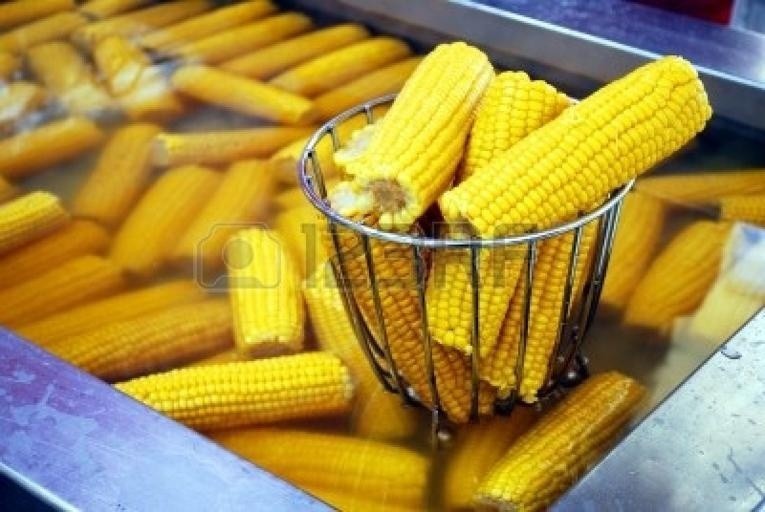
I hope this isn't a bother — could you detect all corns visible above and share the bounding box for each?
[0,1,765,512]
